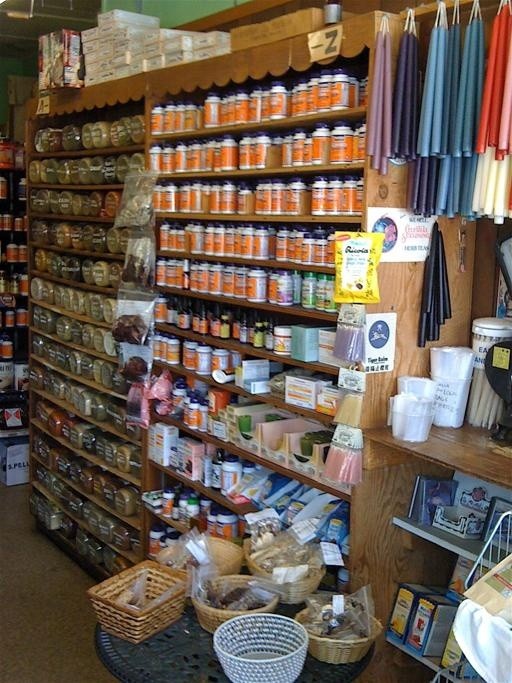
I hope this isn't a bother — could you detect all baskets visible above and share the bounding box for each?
[156,538,243,578]
[213,612,309,682]
[244,534,325,605]
[192,575,279,634]
[296,601,383,664]
[87,560,190,645]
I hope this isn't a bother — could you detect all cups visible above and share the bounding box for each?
[238,415,251,432]
[429,373,476,430]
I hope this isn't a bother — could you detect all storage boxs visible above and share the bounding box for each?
[0,439,30,487]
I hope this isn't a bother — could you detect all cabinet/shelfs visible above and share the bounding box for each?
[27,76,160,586]
[0,164,27,438]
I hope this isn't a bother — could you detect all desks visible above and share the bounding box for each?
[94,566,375,683]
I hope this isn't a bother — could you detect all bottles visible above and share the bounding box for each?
[301,430,335,463]
[0,139,30,395]
[465,316,512,432]
[151,66,370,385]
[171,376,209,434]
[323,0,341,26]
[148,447,262,557]
